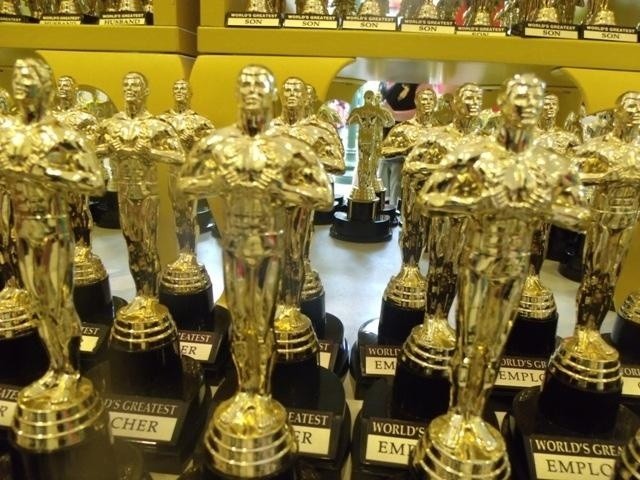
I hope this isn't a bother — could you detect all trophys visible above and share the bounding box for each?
[0,58,640,480]
[225,0,640,43]
[0,0,154,26]
